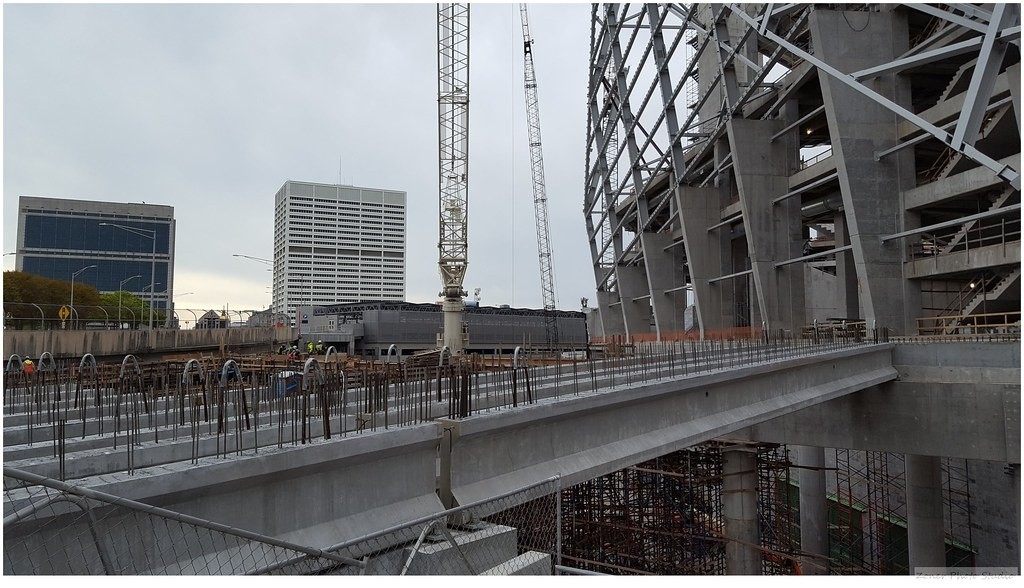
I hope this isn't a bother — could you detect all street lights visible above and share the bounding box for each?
[233,252,280,327]
[100,221,157,329]
[3,252,293,327]
[71,264,94,330]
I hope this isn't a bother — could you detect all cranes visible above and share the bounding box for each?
[519,5,562,354]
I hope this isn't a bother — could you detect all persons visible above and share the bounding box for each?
[23,356,34,379]
[307,341,314,355]
[316,340,326,354]
[278,344,300,358]
[288,350,296,366]
